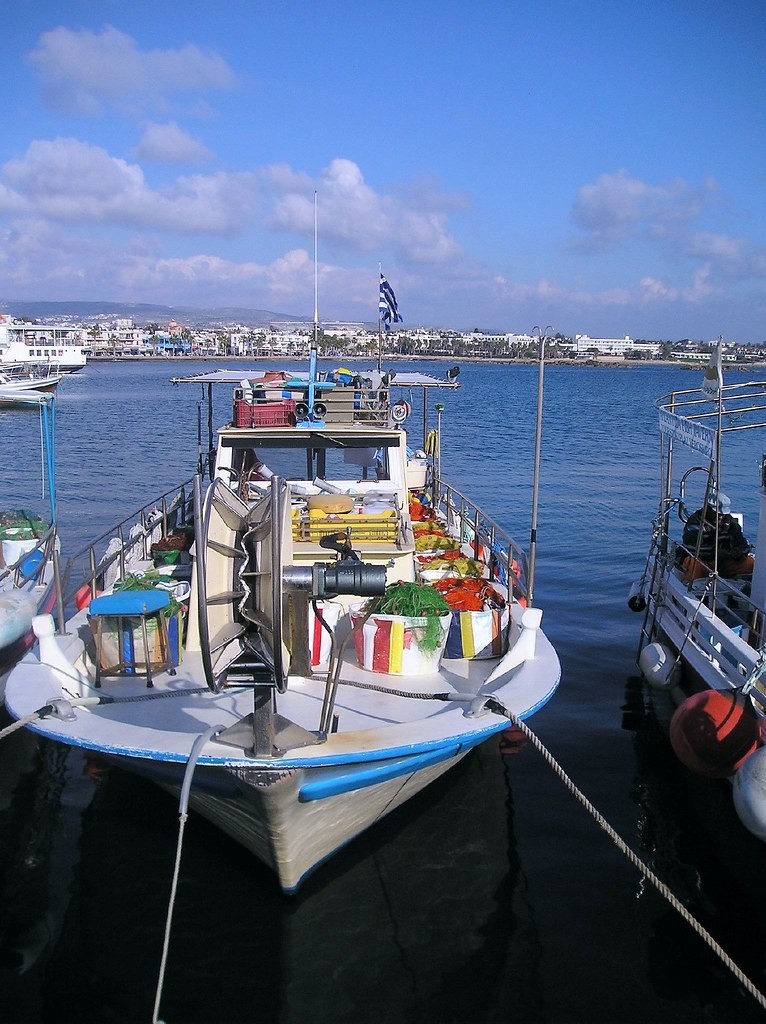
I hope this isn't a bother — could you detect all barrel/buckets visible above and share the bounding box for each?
[19,549,47,581]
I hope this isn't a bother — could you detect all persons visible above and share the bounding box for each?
[683,492,751,562]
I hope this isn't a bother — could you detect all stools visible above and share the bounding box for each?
[90,590,177,689]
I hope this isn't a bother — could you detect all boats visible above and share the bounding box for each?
[0,325,87,374]
[0,360,62,393]
[637,379,766,744]
[2,185,562,898]
[0,386,61,654]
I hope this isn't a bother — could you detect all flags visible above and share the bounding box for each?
[701,342,722,402]
[379,273,402,330]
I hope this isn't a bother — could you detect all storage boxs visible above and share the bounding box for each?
[234,400,296,428]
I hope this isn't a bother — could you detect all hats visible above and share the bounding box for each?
[708,493,731,515]
